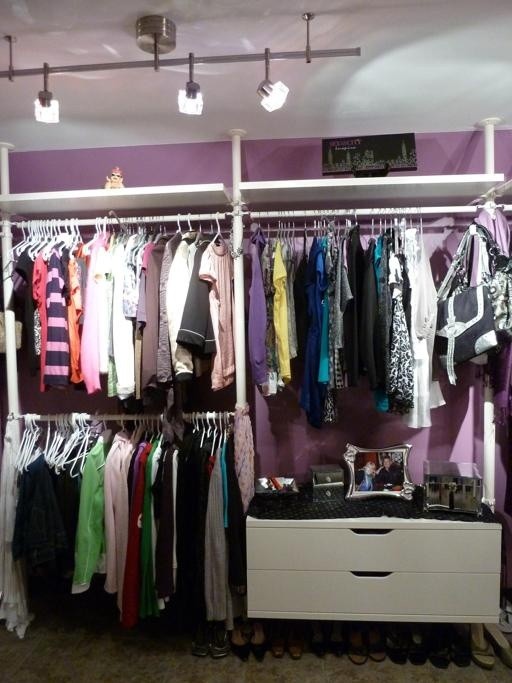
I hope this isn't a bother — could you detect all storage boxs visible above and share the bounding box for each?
[309,462,344,501]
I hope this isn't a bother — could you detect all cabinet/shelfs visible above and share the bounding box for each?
[1,121,508,628]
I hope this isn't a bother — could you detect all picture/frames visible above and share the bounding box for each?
[344,443,416,501]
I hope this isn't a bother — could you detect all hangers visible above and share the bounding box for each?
[12,409,236,481]
[479,191,502,220]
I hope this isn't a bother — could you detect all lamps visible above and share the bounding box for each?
[34,58,292,125]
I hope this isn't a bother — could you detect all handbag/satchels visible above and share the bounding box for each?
[434,255,512,370]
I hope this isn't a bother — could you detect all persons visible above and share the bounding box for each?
[354,460,381,492]
[377,455,401,490]
